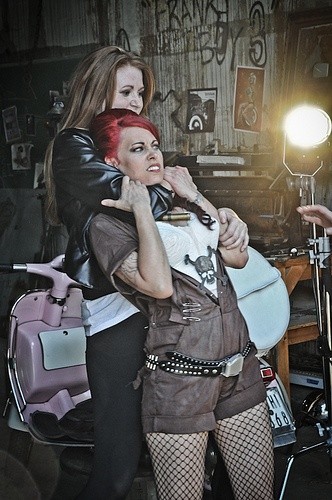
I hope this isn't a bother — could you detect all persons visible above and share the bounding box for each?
[297,204,332,235]
[87,108,275,500]
[42,46,249,500]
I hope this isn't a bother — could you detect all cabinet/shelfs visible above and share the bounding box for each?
[266,251,324,406]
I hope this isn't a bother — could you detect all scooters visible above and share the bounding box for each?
[0,253,297,500]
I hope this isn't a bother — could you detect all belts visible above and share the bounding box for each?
[146,339,253,379]
